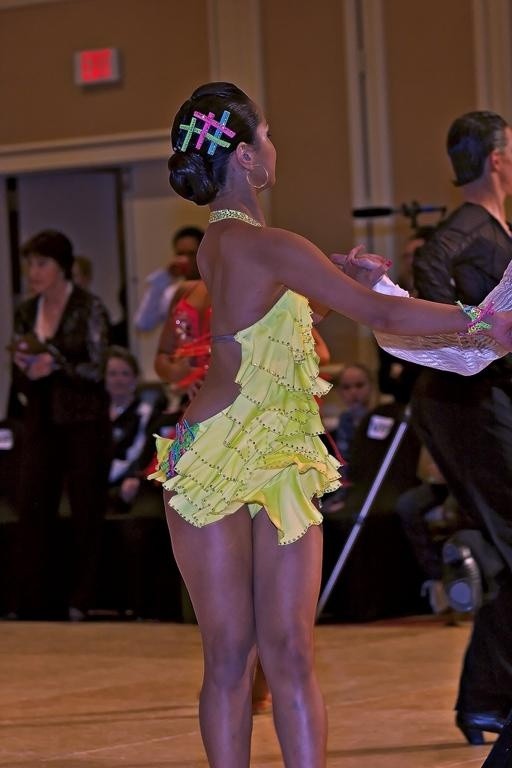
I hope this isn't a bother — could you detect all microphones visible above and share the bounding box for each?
[351,206,446,217]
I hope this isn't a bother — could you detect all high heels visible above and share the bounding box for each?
[452,708,512,746]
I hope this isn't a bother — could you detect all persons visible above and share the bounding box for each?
[407,110,512,746]
[143,81,512,767]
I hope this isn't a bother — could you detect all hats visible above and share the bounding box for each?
[22,229,75,280]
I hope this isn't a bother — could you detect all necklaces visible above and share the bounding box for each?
[204,207,263,227]
